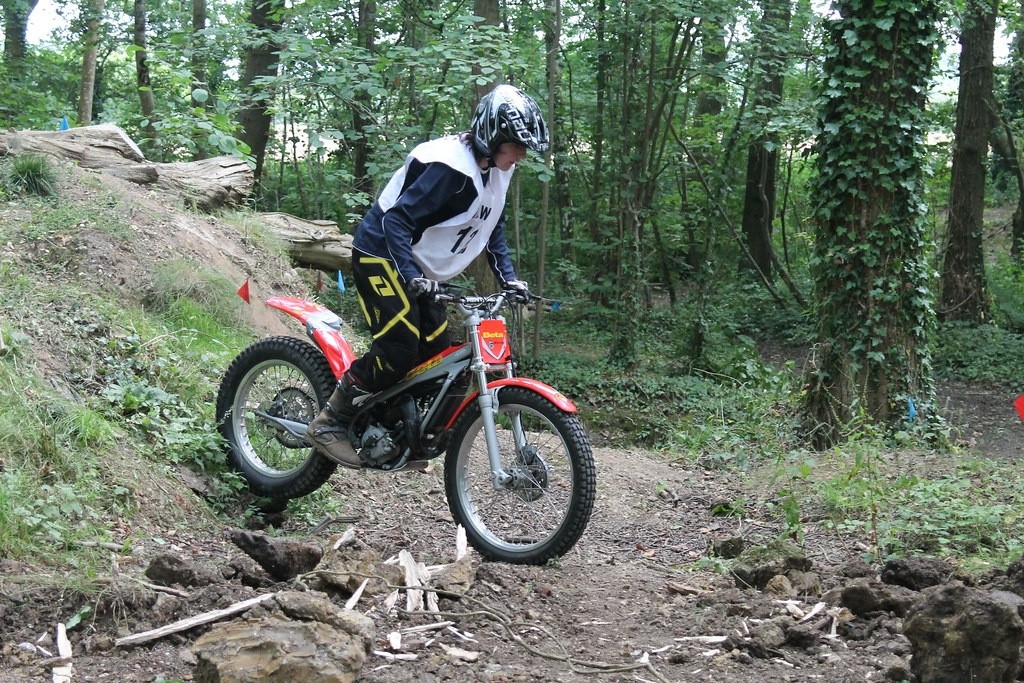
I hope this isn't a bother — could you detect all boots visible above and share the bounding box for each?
[303,370,372,469]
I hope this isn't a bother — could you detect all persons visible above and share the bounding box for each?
[301,83,552,469]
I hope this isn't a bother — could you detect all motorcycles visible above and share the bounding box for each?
[214,287,597,566]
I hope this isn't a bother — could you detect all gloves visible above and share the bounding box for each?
[505,279,529,304]
[410,277,439,298]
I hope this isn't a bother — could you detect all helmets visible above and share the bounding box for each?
[471,83,549,156]
